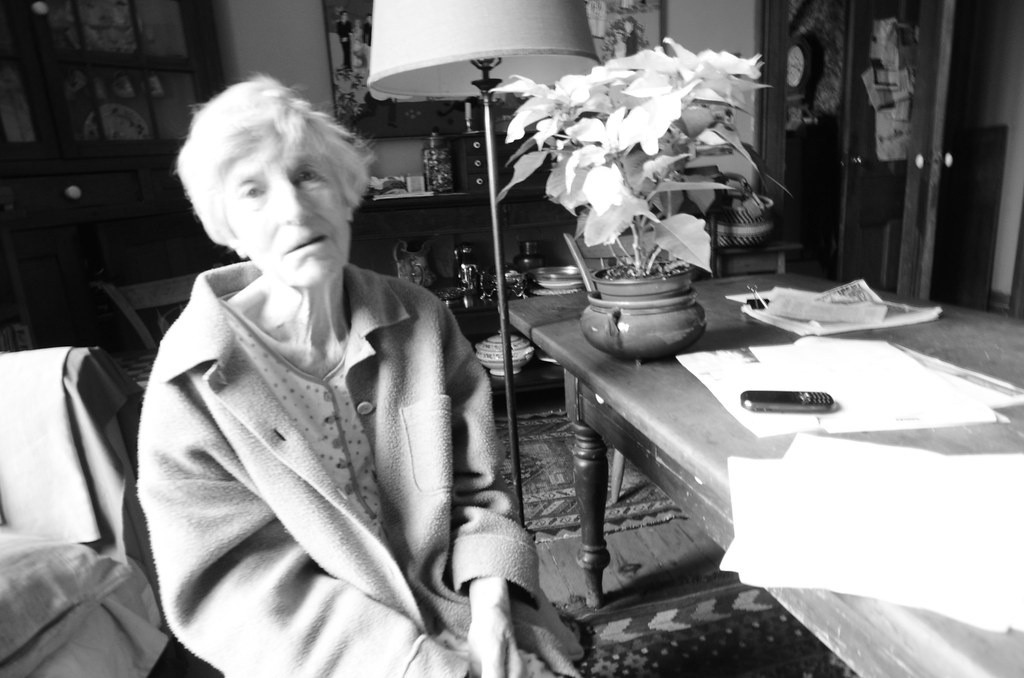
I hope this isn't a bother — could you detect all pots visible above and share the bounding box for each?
[474,329,535,376]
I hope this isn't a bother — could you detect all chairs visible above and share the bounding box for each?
[563,232,655,291]
[715,172,795,278]
[88,263,223,354]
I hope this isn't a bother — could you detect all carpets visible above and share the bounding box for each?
[496,407,689,544]
[562,582,858,678]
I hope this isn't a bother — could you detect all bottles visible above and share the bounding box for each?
[422,128,455,194]
[512,241,543,274]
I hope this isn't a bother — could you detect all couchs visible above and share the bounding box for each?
[0,343,170,678]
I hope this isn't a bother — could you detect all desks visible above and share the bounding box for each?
[498,272,1024,678]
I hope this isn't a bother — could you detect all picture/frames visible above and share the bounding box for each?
[322,0,667,142]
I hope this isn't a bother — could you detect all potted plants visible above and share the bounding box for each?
[485,36,774,359]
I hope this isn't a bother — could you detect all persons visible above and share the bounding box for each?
[614,18,637,58]
[138,74,584,678]
[337,11,372,71]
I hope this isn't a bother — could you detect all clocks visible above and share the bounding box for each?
[786,29,825,92]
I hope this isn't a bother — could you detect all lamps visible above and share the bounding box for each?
[368,0,598,526]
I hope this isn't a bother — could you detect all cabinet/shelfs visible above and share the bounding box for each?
[0,0,228,350]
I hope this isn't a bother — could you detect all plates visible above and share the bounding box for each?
[539,282,584,290]
[529,265,596,282]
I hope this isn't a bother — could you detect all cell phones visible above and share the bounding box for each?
[741,390,836,412]
